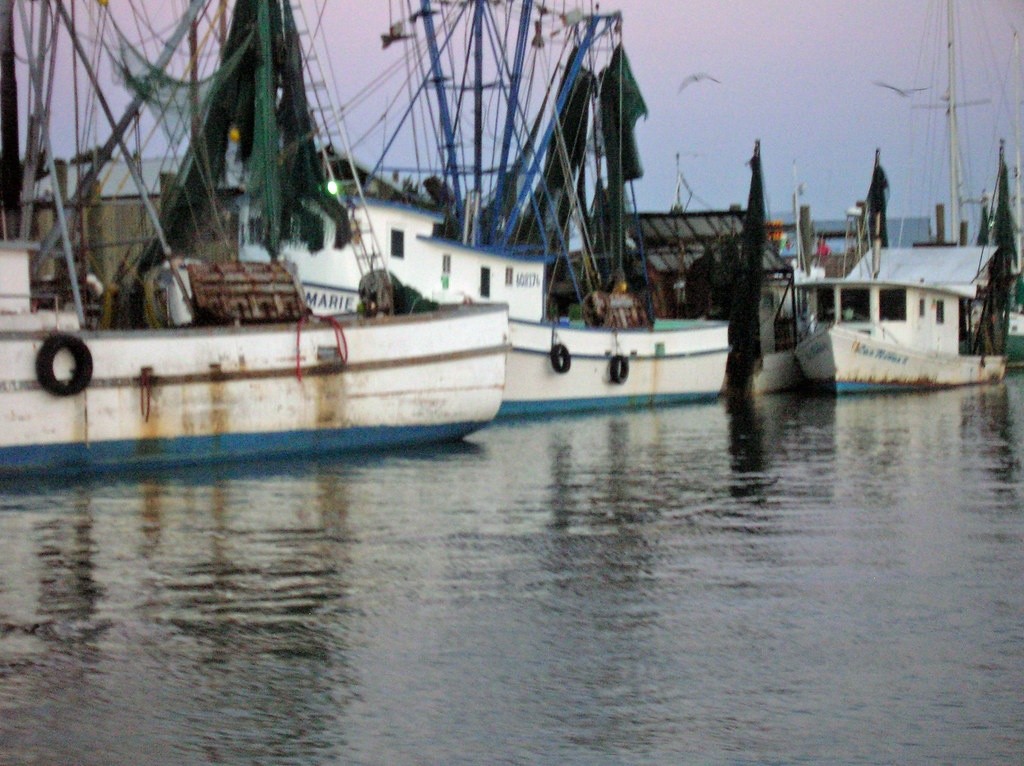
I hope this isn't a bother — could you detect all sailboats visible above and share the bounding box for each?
[0,0,1024,479]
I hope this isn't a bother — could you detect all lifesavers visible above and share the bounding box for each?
[36,336,93,399]
[550,344,571,374]
[610,355,628,385]
